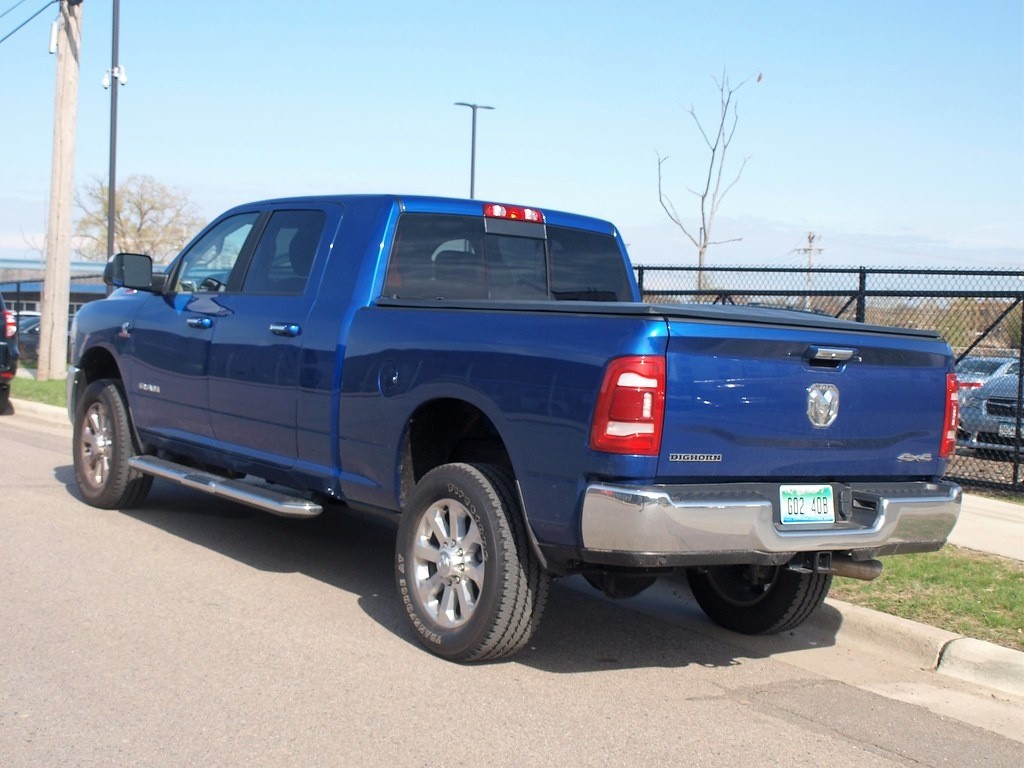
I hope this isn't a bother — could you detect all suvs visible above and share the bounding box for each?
[0,293,20,412]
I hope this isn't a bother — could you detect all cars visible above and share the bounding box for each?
[955,356,1020,392]
[961,372,1024,455]
[17,316,72,358]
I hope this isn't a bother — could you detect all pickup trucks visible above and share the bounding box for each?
[68,193,961,665]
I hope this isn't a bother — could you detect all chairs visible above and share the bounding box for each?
[435,249,477,299]
[276,229,320,292]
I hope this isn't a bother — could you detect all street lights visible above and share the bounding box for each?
[455,101,495,253]
